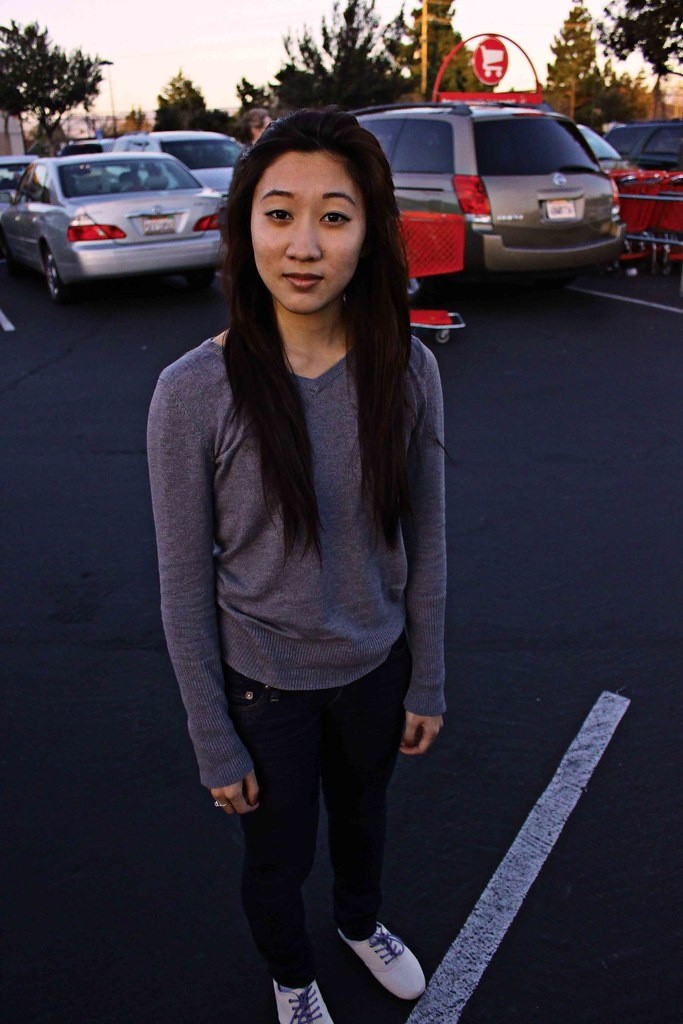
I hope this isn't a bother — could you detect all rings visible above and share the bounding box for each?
[215,800,230,807]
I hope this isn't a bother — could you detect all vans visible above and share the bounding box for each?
[112,130,249,207]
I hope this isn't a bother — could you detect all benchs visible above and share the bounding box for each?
[76,177,168,194]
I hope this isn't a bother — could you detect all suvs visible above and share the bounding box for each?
[331,100,628,285]
[573,116,683,180]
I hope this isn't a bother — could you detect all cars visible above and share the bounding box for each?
[0,155,42,215]
[55,138,116,157]
[0,151,233,306]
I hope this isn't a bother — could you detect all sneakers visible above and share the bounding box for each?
[273,975,334,1024]
[338,918,427,1000]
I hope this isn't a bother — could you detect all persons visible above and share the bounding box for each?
[148,109,446,1024]
